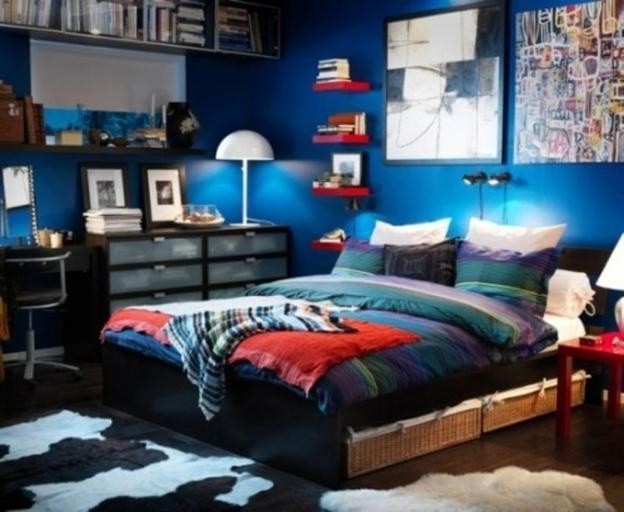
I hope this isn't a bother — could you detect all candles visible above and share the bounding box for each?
[161,104,167,125]
[151,88,156,117]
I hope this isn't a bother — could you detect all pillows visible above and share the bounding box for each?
[329,240,384,279]
[383,238,460,288]
[539,264,594,323]
[456,241,556,314]
[369,219,453,251]
[463,219,566,256]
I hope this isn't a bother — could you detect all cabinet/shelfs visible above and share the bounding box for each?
[0,0,283,156]
[77,224,293,363]
[311,82,372,249]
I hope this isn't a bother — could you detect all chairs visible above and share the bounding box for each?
[0,246,83,384]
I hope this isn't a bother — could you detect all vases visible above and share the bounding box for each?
[166,100,195,150]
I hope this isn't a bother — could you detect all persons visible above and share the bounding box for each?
[97,181,109,208]
[157,181,173,203]
[104,181,116,208]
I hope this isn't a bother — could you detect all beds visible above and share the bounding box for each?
[99,240,612,493]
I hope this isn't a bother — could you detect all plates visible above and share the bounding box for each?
[174,216,225,229]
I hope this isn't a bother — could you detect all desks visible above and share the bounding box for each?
[0,244,94,276]
[558,331,623,459]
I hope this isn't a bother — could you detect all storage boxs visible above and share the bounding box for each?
[0,97,26,143]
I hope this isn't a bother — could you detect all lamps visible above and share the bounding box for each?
[486,171,512,225]
[595,235,624,349]
[463,171,490,222]
[215,131,276,229]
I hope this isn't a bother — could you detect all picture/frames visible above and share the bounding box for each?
[77,163,129,212]
[138,162,188,231]
[383,2,509,167]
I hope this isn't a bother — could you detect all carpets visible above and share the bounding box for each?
[319,462,621,511]
[0,402,334,511]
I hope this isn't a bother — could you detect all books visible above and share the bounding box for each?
[0,0,266,54]
[316,111,365,136]
[315,58,352,82]
[23,95,46,146]
[82,206,144,234]
